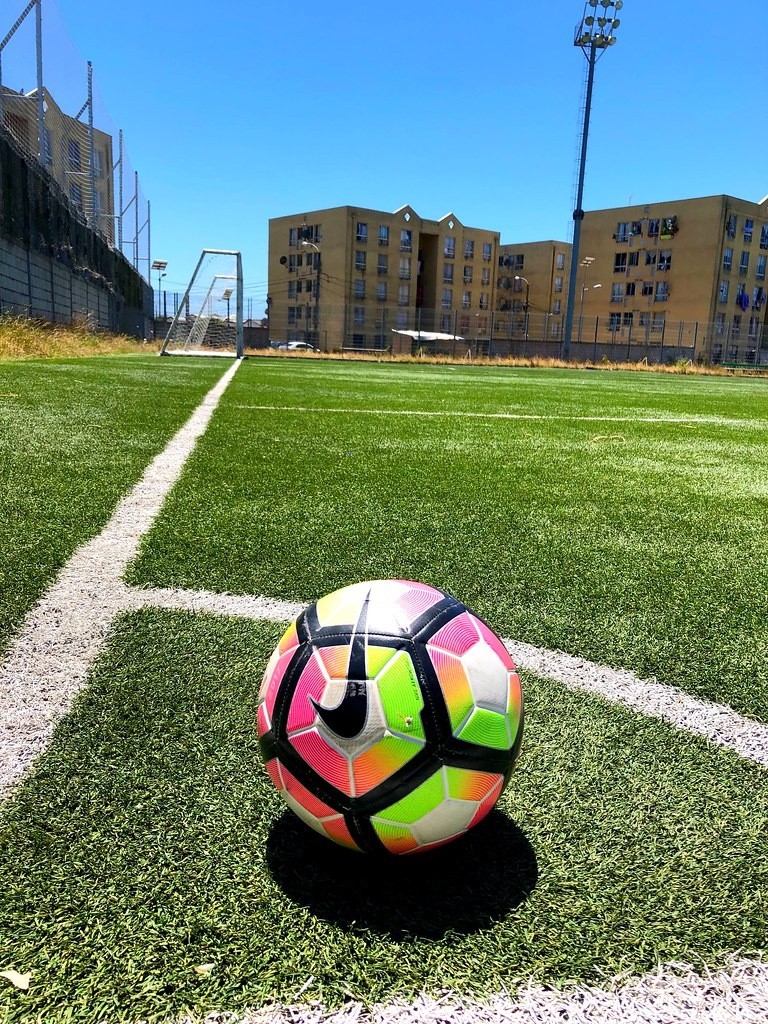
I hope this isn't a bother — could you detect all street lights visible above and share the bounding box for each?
[302,240,320,352]
[560,0,623,361]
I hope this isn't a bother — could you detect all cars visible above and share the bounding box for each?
[278,340,320,353]
[269,338,286,349]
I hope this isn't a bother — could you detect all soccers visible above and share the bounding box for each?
[256,578,523,856]
